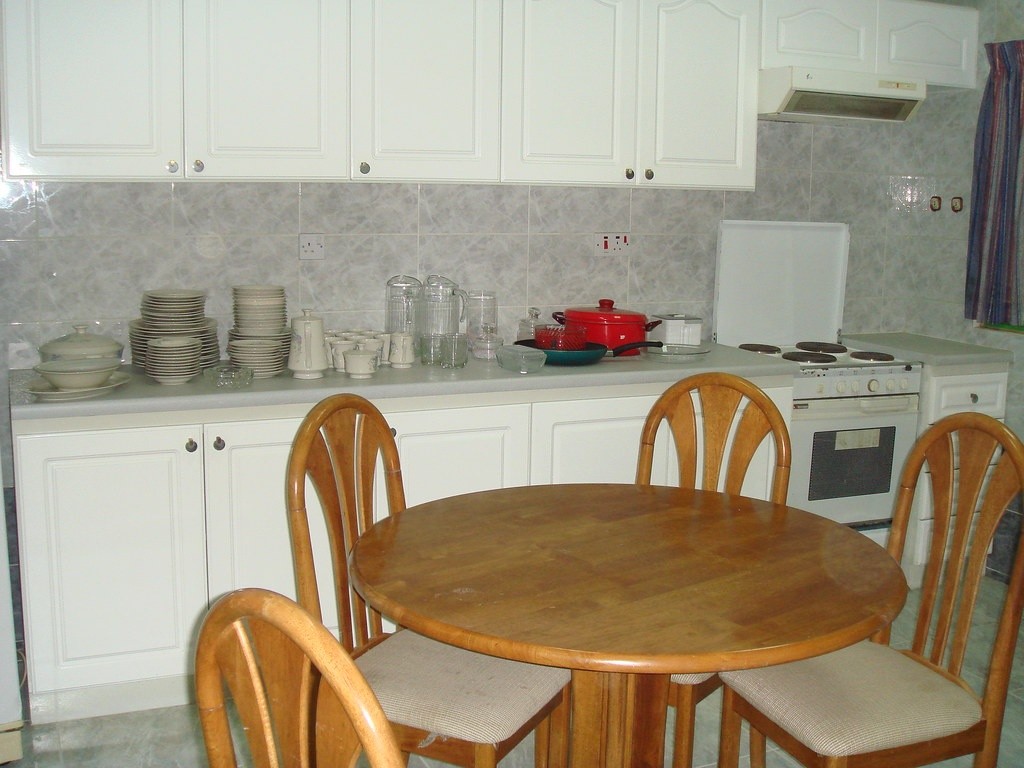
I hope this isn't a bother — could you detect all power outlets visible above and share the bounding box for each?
[299,233,325,260]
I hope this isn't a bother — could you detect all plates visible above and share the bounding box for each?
[225,283,293,381]
[18,371,132,400]
[128,288,221,386]
[637,344,712,364]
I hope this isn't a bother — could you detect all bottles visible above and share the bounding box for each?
[516,307,547,341]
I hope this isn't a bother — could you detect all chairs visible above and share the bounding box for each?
[192,588,408,768]
[717,410,1024,768]
[287,392,571,768]
[635,372,792,768]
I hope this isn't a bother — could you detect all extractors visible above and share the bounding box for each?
[757,66,927,126]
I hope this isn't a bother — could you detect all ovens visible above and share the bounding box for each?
[786,394,920,527]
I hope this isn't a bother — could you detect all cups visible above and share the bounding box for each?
[420,333,468,370]
[468,333,505,361]
[324,327,415,379]
[465,290,498,351]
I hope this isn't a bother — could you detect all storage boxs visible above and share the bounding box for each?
[593,232,630,257]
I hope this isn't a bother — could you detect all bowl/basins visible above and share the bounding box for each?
[534,324,589,351]
[495,344,547,373]
[33,357,121,390]
[203,364,254,390]
[37,325,124,362]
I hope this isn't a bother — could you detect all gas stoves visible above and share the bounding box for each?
[738,342,922,399]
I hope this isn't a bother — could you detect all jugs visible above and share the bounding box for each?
[287,308,326,379]
[384,274,421,357]
[421,275,470,334]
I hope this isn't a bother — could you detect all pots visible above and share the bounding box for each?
[513,338,663,366]
[552,299,663,357]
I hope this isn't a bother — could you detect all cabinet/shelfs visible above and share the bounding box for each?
[896,362,1010,591]
[12,376,794,723]
[0,1,763,190]
[762,0,979,89]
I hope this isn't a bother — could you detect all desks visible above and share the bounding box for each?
[349,483,908,768]
